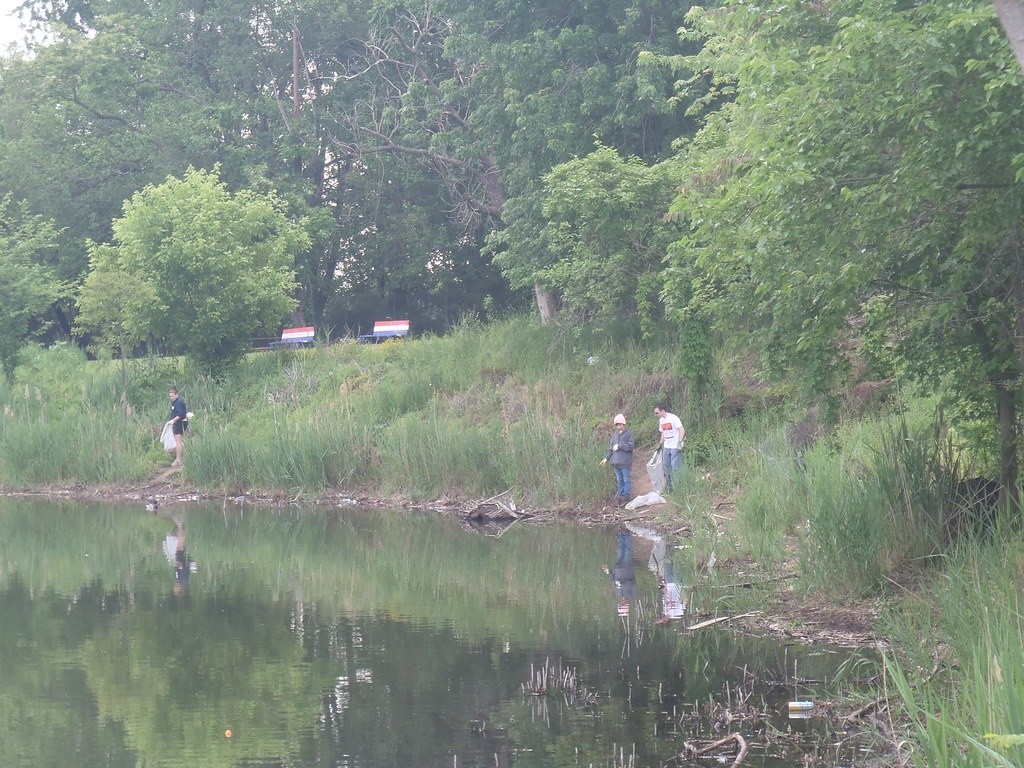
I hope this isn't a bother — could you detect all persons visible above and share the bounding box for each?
[599,414,635,509]
[167,386,188,465]
[603,528,688,629]
[654,403,686,493]
[171,510,188,597]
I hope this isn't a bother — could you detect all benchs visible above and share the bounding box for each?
[269,327,316,348]
[360,320,412,343]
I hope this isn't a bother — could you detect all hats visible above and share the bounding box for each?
[614,413,626,425]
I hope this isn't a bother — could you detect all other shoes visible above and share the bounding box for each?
[614,495,631,508]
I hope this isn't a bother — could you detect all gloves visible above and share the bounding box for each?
[654,443,662,453]
[599,459,607,467]
[676,441,683,450]
[612,444,618,452]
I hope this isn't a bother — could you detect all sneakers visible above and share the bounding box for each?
[170,459,184,467]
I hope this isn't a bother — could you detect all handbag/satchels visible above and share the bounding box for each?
[179,418,189,432]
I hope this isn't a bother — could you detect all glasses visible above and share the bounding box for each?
[655,411,661,415]
